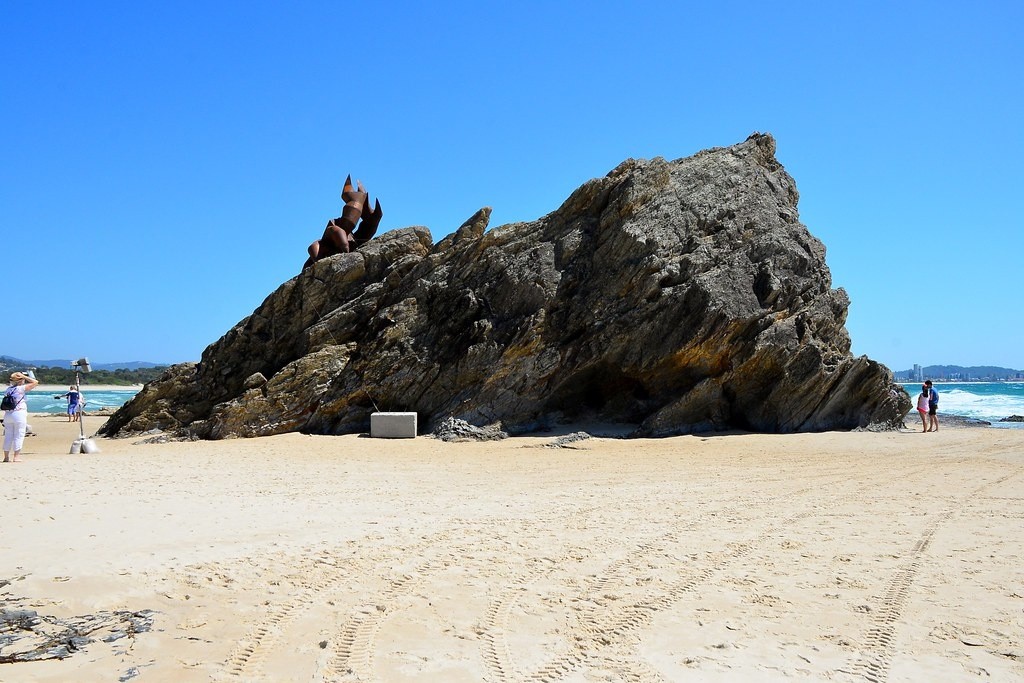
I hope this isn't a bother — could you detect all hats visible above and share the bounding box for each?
[10,372,23,382]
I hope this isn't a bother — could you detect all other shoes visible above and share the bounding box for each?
[3,459,9,462]
[13,459,22,462]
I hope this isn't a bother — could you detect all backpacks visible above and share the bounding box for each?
[1,388,24,410]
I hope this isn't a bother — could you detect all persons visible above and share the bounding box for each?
[3,372,38,463]
[917,384,930,433]
[62,386,84,422]
[925,380,939,432]
